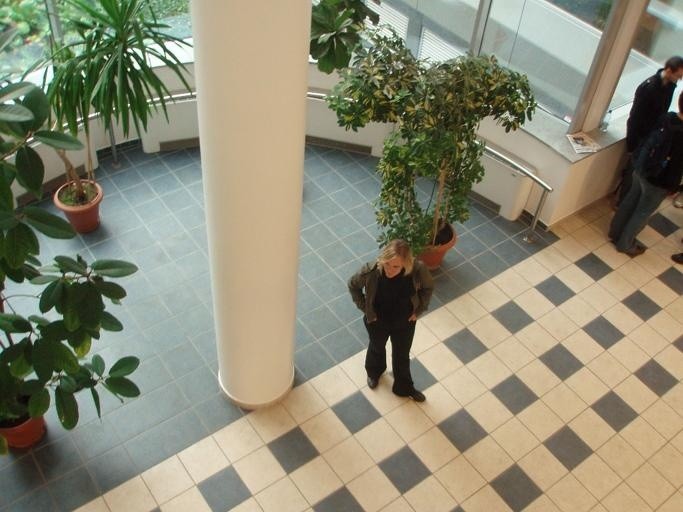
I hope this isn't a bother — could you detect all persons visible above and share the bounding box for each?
[347,238,433,405]
[607,88,682,255]
[611,56,682,215]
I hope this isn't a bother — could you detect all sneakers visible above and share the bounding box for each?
[367,376,377,388]
[408,388,425,402]
[626,245,645,256]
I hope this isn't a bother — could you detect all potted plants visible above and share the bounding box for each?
[18,0,196,235]
[0,80,141,451]
[308,0,539,271]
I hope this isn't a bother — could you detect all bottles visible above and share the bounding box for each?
[600,109,612,132]
